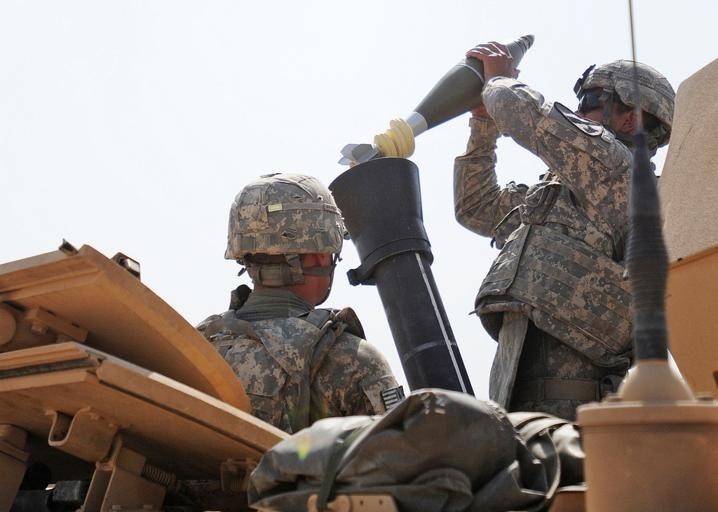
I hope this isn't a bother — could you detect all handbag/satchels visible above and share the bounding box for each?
[244,387,587,512]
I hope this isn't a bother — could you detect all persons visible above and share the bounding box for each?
[462,38,679,423]
[190,170,412,433]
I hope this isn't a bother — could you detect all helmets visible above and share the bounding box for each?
[223,171,351,261]
[573,58,677,148]
